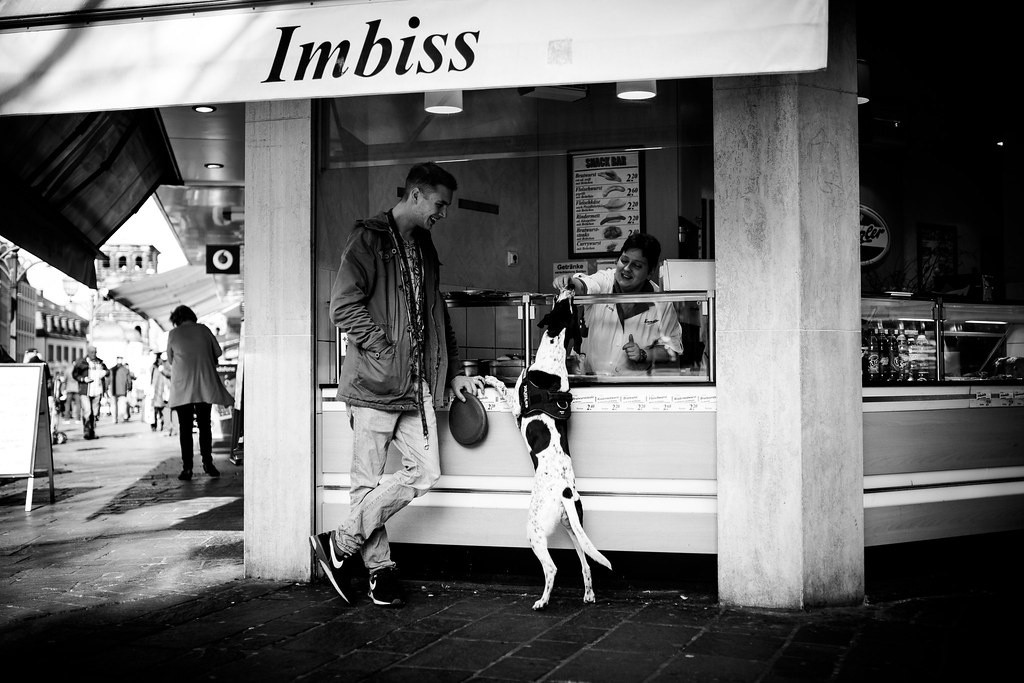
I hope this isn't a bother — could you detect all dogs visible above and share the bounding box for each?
[479,279,613,612]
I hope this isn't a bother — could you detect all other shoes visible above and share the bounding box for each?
[178,469,193,480]
[202,463,220,477]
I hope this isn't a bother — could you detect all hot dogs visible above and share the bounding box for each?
[600,213,626,225]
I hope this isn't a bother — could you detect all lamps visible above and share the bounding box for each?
[424,90,462,114]
[857,58,872,106]
[616,80,657,101]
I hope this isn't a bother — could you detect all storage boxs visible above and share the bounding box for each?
[658,257,715,292]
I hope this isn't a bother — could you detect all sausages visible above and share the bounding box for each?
[602,185,625,197]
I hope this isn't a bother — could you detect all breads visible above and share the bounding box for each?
[604,199,626,210]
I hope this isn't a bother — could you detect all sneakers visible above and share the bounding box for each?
[309,531,354,605]
[367,566,404,605]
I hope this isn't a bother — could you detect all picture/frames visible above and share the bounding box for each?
[567,147,646,259]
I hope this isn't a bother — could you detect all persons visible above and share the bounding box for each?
[166,305,235,481]
[309,162,486,606]
[27,346,177,445]
[550,232,683,373]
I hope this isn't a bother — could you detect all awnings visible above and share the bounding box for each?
[0,107,226,334]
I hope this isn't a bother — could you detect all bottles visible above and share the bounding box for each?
[896,329,909,380]
[867,328,878,379]
[886,328,898,380]
[915,330,930,381]
[878,328,889,380]
[908,334,918,381]
